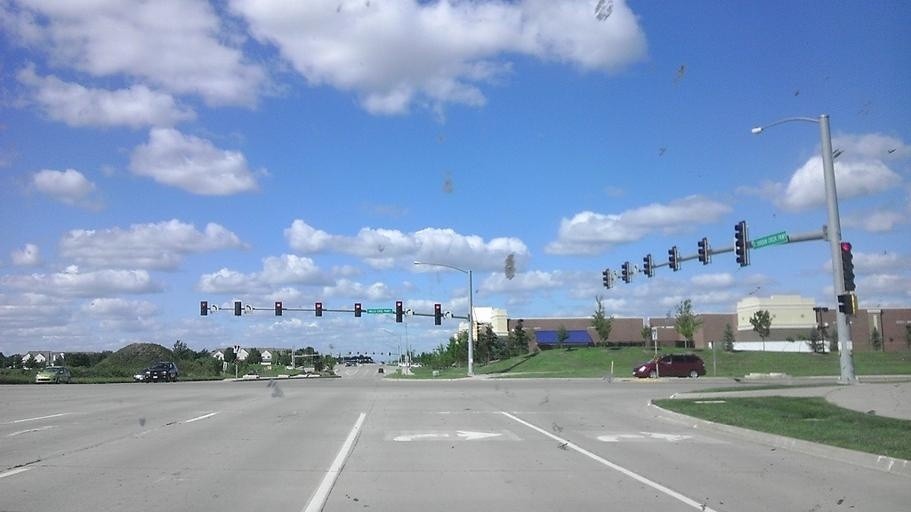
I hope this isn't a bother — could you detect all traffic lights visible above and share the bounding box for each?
[354,304,361,316]
[734,221,749,267]
[644,254,652,276]
[315,303,321,316]
[621,261,629,283]
[396,301,401,323]
[603,268,612,289]
[434,304,441,325]
[668,247,677,272]
[837,295,852,315]
[697,238,707,264]
[275,302,282,316]
[841,243,854,290]
[234,302,240,315]
[200,301,207,315]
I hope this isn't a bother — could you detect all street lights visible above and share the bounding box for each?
[414,261,473,376]
[812,307,828,353]
[751,115,856,383]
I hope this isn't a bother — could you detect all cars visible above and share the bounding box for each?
[34,365,69,384]
[134,362,178,383]
[632,353,704,377]
